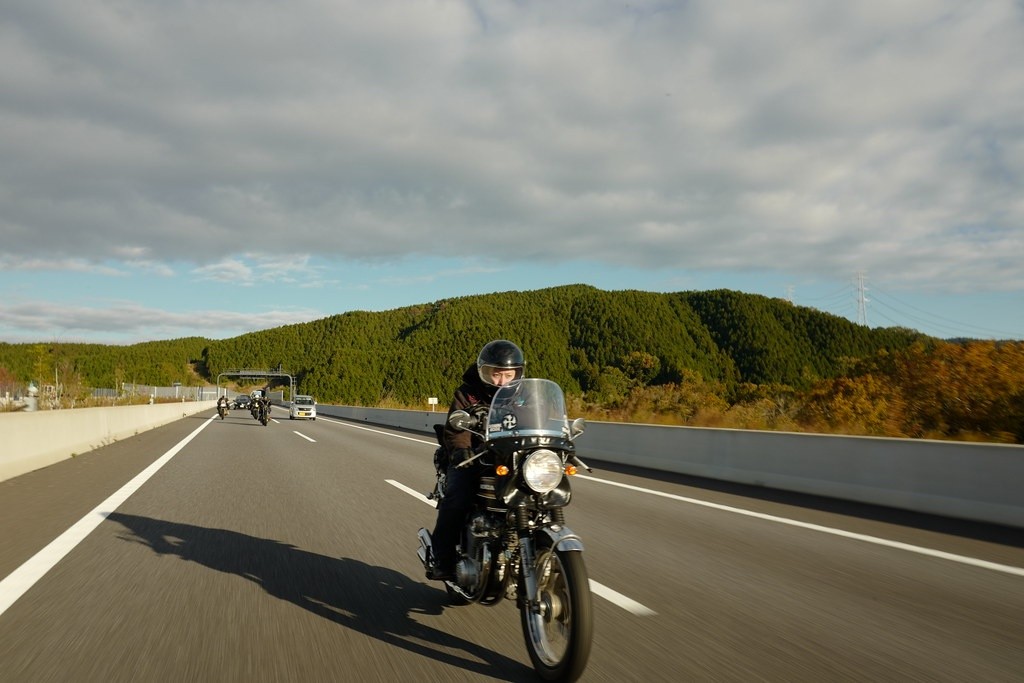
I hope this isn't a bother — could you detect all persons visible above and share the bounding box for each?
[251,395,261,415]
[432,339,549,580]
[259,396,272,419]
[217,395,230,413]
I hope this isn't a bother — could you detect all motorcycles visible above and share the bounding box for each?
[217,399,229,420]
[417,378,593,682]
[251,398,259,420]
[259,400,272,426]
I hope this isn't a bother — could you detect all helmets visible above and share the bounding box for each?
[473,340,524,405]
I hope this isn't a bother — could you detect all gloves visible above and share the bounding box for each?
[451,449,474,473]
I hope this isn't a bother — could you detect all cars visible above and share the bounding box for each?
[238,395,252,410]
[289,395,317,421]
[233,396,239,410]
[247,397,271,409]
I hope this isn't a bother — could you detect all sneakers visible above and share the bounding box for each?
[430,548,450,577]
